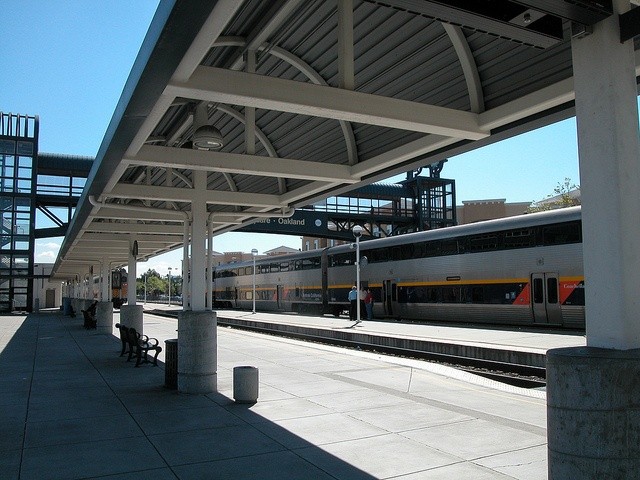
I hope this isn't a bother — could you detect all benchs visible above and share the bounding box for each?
[115,321,163,369]
[80,307,98,330]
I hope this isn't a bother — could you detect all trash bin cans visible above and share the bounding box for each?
[165,339,177,390]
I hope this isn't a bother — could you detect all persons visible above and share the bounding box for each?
[349,286,358,320]
[359,287,368,320]
[365,289,374,320]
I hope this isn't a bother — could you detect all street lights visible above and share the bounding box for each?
[353,225,363,328]
[168,267,172,307]
[251,248,258,315]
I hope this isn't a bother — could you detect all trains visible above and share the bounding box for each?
[181,203,586,330]
[66,268,128,309]
